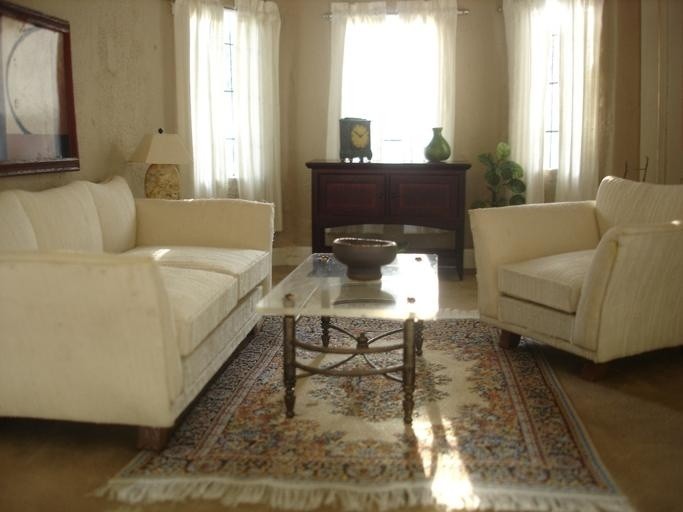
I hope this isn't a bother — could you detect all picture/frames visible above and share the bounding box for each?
[1,0,82,177]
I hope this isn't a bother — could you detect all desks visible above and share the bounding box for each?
[304,158,472,281]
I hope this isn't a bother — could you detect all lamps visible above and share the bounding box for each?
[144,129,191,199]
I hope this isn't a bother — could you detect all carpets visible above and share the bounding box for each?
[95,310,638,511]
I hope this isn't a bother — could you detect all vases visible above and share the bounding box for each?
[424,126,451,161]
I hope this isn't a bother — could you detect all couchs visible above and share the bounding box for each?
[1,173,276,452]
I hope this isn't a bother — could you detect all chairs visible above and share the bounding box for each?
[466,173,683,385]
